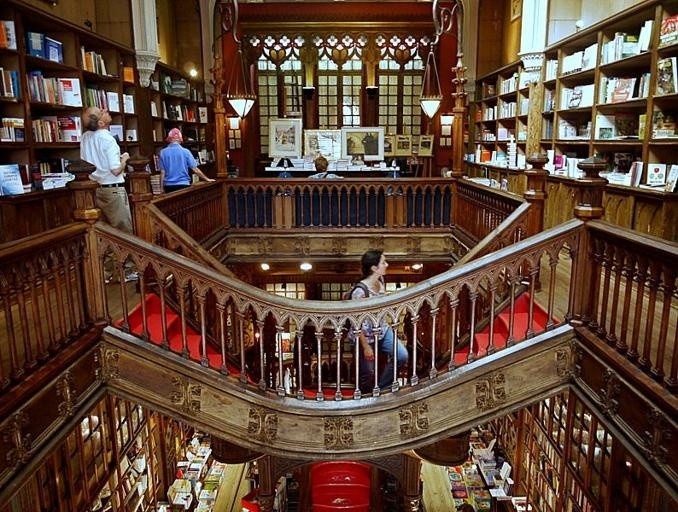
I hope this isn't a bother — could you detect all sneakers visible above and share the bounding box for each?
[118,272,139,283]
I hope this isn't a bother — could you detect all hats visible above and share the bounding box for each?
[165,128,184,145]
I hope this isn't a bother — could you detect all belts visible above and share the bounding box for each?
[102,183,125,188]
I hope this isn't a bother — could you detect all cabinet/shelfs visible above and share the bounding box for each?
[146,59,218,191]
[472,57,533,196]
[0,0,146,244]
[447,388,677,512]
[533,0,677,252]
[0,395,226,512]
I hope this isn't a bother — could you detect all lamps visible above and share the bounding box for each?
[418,0,468,121]
[213,0,259,117]
[300,86,316,100]
[365,84,377,101]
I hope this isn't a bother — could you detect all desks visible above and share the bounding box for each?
[263,167,402,179]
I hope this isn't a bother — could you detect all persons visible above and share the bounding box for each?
[346,248,411,394]
[307,156,345,181]
[158,128,217,194]
[78,103,143,285]
[351,155,364,165]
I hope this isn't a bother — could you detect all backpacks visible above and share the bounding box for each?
[343,276,384,335]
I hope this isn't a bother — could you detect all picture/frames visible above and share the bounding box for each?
[342,126,385,163]
[268,117,303,160]
[303,127,341,161]
[509,0,521,21]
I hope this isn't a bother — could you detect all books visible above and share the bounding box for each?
[538,14,677,193]
[443,397,614,511]
[81,413,265,511]
[462,69,528,189]
[347,164,368,169]
[270,156,350,168]
[0,16,211,197]
